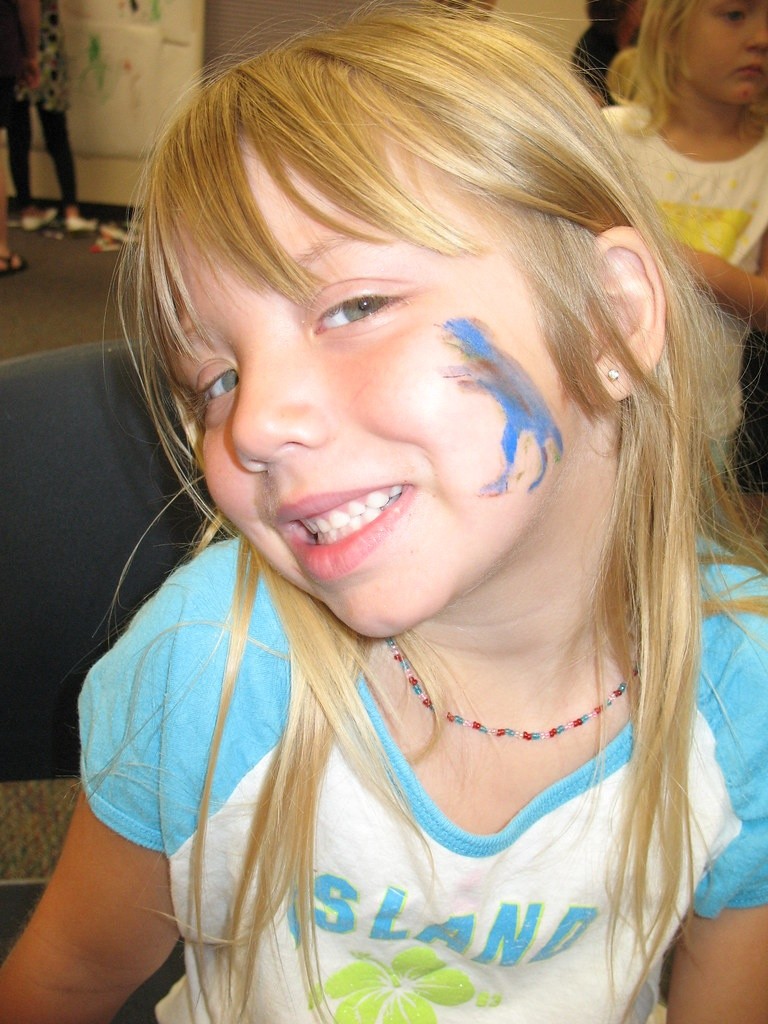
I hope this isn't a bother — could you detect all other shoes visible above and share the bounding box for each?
[21,208,57,231]
[66,216,97,231]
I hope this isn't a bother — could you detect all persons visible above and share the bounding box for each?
[0,0,767,1024]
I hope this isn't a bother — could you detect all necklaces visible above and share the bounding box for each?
[387,638,639,741]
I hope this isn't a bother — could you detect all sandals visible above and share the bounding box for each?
[0,252,27,275]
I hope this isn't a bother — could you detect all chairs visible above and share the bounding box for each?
[0,338,231,1024]
